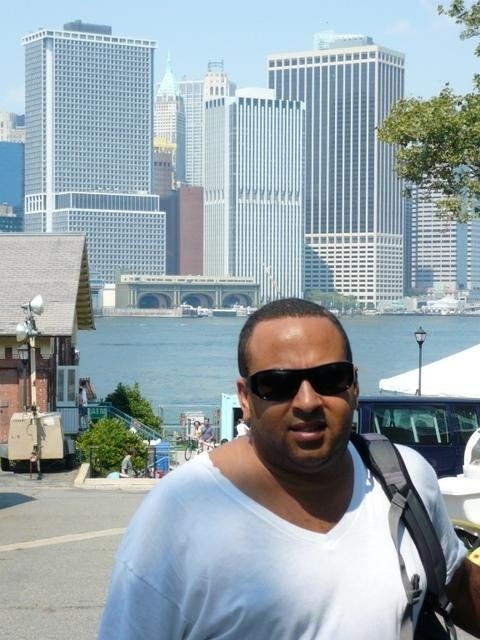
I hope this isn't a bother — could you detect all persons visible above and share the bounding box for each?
[193,421,203,439]
[81,381,89,424]
[95,297,479,639]
[79,388,88,430]
[220,438,228,445]
[236,418,249,438]
[128,420,141,436]
[200,417,214,452]
[122,448,136,478]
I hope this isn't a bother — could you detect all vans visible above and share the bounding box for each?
[349,393,480,475]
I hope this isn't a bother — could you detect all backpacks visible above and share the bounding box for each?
[349,432,458,640]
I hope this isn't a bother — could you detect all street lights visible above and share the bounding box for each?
[6,294,67,473]
[414,326,426,395]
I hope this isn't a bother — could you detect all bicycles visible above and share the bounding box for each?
[184,435,215,462]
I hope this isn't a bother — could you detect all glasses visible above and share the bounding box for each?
[245,360,355,400]
